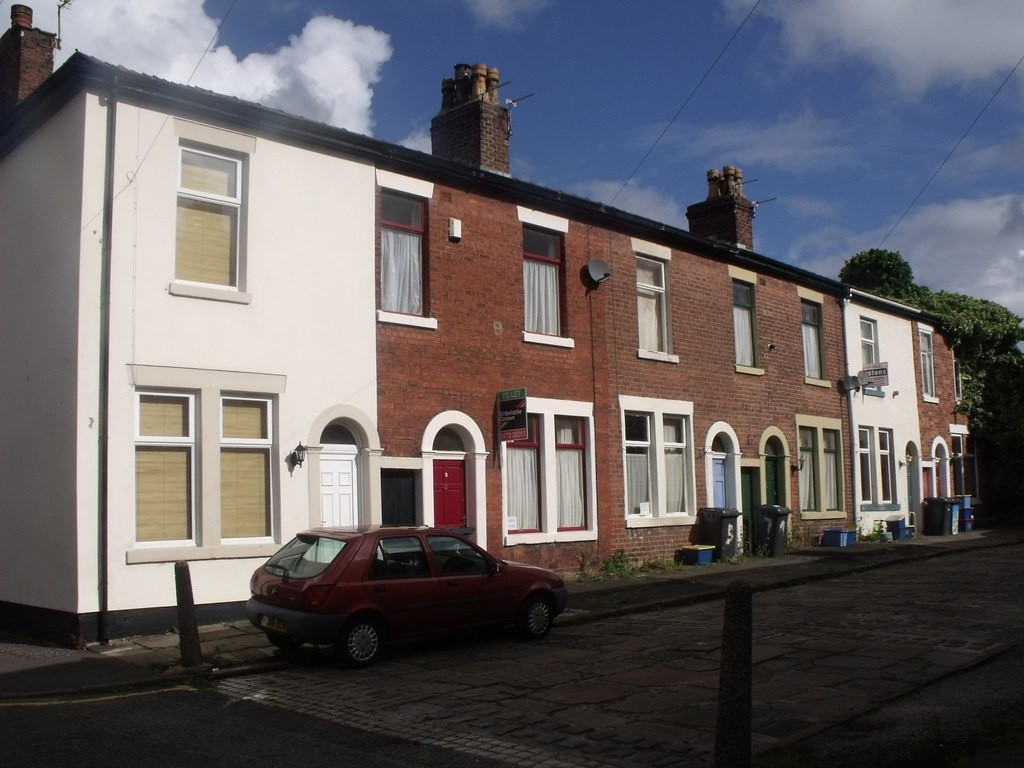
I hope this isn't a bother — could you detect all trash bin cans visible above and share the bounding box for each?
[682,545,716,565]
[923,497,961,536]
[699,507,743,561]
[755,505,792,558]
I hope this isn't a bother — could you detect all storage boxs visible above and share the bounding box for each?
[885,515,915,540]
[955,495,975,533]
[823,529,857,547]
[681,545,716,565]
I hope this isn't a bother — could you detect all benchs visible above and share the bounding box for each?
[295,559,331,574]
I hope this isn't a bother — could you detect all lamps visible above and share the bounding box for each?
[791,454,806,476]
[899,455,913,470]
[291,442,308,469]
[950,450,958,466]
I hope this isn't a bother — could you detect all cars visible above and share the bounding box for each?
[246,526,569,670]
